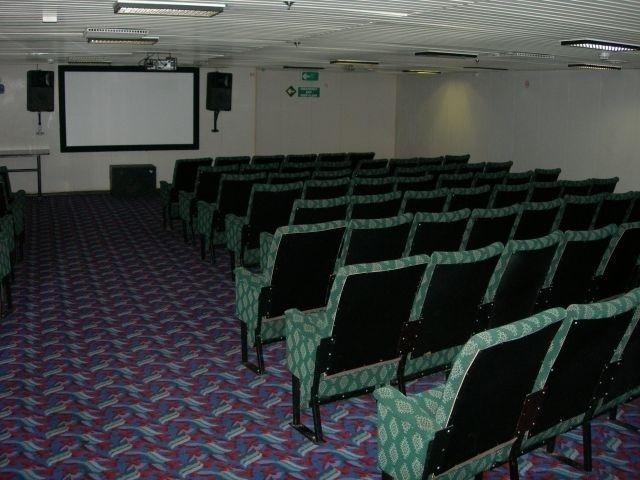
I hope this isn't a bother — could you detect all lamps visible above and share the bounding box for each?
[88,37,159,45]
[112,2,225,18]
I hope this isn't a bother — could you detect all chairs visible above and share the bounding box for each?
[282,252,431,447]
[372,306,567,478]
[566,288,640,479]
[396,241,504,404]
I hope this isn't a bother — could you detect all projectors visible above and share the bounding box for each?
[145,58,177,72]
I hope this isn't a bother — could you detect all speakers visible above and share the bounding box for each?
[27,70,54,112]
[206,72,233,111]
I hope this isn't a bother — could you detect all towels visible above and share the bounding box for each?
[560,39,638,53]
